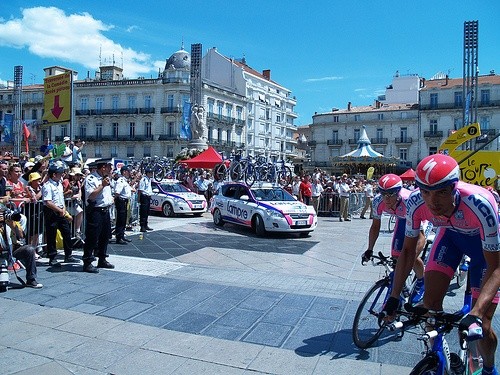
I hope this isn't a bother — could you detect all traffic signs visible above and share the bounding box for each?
[42,70,71,126]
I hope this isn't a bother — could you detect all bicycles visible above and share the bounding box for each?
[129,156,189,183]
[412,240,467,287]
[379,302,484,375]
[388,214,397,233]
[351,251,429,348]
[213,151,292,187]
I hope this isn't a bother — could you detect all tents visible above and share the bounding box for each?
[178,145,231,173]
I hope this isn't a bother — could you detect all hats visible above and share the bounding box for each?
[35,155,45,164]
[95,157,112,165]
[28,172,42,183]
[87,162,97,169]
[49,164,65,173]
[120,166,128,172]
[145,168,153,174]
[24,162,35,170]
[63,137,70,142]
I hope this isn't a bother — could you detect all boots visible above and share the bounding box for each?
[97,256,114,268]
[82,261,100,273]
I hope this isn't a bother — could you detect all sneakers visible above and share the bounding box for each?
[411,280,426,302]
[26,278,43,288]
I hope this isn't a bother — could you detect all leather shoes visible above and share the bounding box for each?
[65,254,80,263]
[122,236,132,242]
[49,257,62,269]
[116,237,128,245]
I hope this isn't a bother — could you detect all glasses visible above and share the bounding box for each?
[380,193,396,198]
[344,179,347,180]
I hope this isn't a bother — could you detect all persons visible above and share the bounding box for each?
[114,165,132,245]
[83,157,115,274]
[139,168,154,232]
[0,204,44,291]
[362,173,432,318]
[42,164,80,266]
[492,175,500,195]
[485,186,500,207]
[276,166,419,222]
[0,136,144,259]
[382,152,500,375]
[180,167,226,208]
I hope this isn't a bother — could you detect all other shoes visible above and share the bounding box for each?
[340,216,374,222]
[482,367,497,375]
[140,225,153,232]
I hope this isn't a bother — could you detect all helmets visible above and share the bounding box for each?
[414,154,460,191]
[486,186,493,193]
[377,174,403,193]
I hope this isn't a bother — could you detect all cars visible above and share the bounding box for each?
[210,180,317,238]
[149,177,207,217]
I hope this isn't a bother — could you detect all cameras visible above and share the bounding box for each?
[1,210,22,223]
